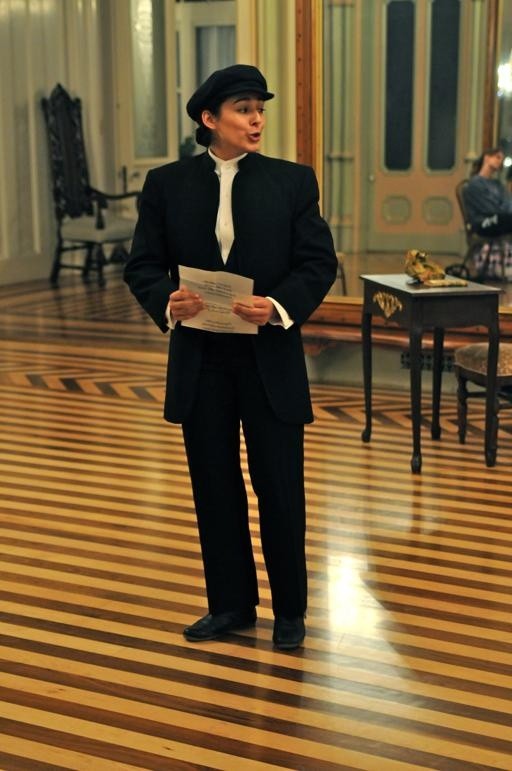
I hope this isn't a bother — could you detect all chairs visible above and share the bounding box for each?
[453,175,512,281]
[42,85,142,287]
[452,341,512,446]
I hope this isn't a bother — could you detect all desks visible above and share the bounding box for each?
[359,272,505,473]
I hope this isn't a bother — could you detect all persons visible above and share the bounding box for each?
[123,59,340,650]
[462,144,512,283]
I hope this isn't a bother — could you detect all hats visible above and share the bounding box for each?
[186,65,274,126]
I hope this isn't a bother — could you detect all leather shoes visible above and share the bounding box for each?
[183,607,257,641]
[273,615,304,648]
